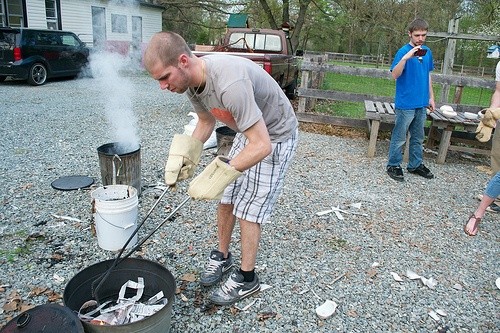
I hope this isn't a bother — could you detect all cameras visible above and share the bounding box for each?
[415,48,427,56]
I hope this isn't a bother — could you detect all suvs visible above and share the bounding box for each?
[0,26,93,87]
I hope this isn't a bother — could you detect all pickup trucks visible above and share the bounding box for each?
[190,28,304,100]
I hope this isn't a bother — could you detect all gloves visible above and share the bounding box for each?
[475,122,493,143]
[164,134,204,185]
[481,108,500,128]
[189,155,244,203]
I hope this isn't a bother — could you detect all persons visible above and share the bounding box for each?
[386,18,435,181]
[144,32,299,305]
[464,61,500,236]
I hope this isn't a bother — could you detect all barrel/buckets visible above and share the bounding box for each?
[62,257,176,333]
[91,185,139,251]
[97,142,142,195]
[216,126,236,157]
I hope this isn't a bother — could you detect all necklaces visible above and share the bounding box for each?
[181,59,204,109]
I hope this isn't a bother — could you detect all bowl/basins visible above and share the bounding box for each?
[464,112,477,119]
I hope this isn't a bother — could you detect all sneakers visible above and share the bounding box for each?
[408,164,434,179]
[210,269,261,305]
[387,165,404,181]
[199,250,233,285]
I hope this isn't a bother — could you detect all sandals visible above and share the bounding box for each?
[464,214,481,236]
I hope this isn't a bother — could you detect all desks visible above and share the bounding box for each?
[365,100,494,164]
